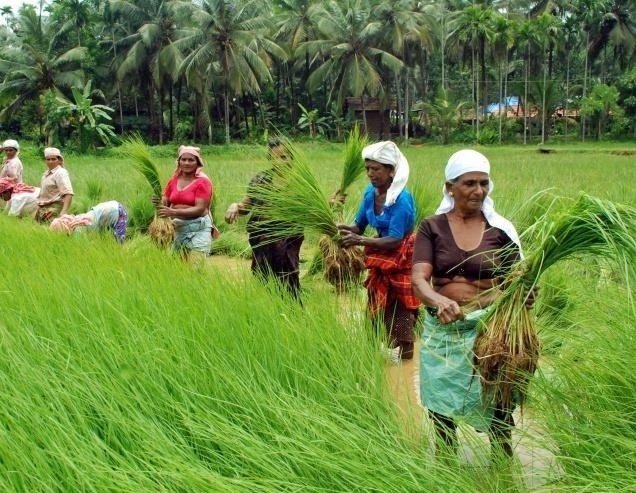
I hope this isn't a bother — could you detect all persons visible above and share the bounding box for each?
[151,144,221,263]
[410,149,540,467]
[49,200,128,245]
[0,139,23,183]
[34,146,75,225]
[336,137,423,360]
[0,178,41,219]
[223,137,348,307]
